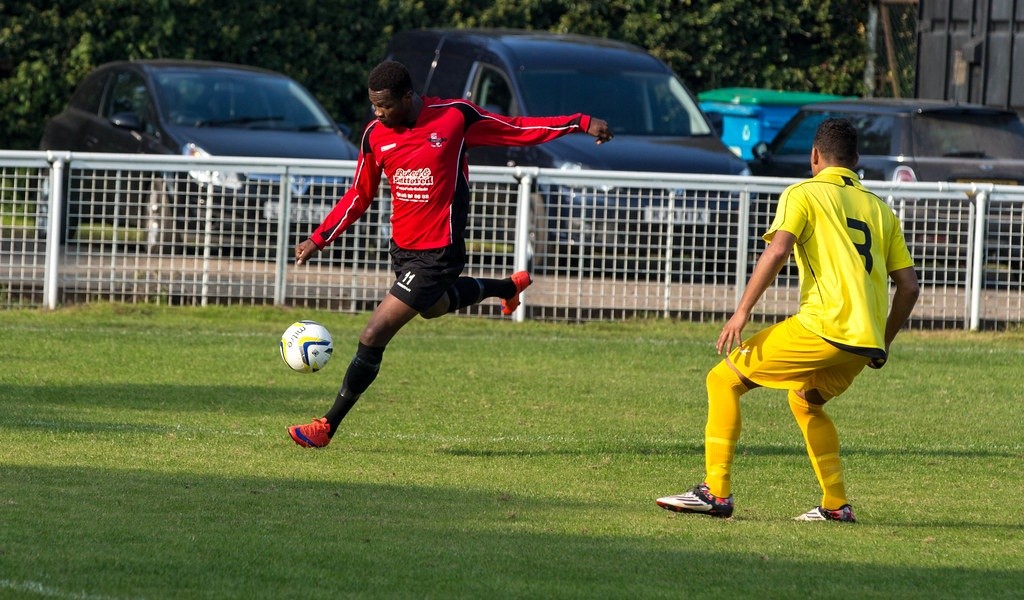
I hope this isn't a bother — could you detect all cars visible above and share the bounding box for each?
[663,86,1024,322]
[32,62,373,258]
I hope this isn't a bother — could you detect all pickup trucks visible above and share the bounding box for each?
[386,28,753,274]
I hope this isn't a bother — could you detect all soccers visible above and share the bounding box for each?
[280,320,334,373]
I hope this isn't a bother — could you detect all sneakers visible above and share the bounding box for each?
[655,482,734,518]
[791,503,855,522]
[499,271,534,315]
[287,416,331,448]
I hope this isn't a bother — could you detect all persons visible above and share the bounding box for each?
[288,62,613,448]
[656,117,920,523]
